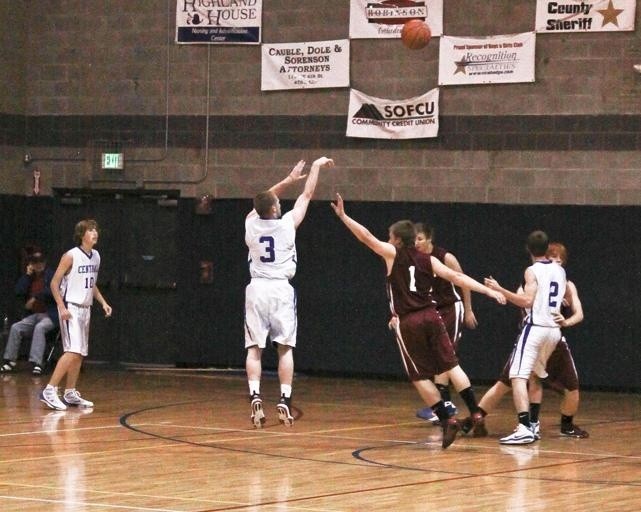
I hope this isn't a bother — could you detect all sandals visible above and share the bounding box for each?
[1,362,19,374]
[30,364,45,377]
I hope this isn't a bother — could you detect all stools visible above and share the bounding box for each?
[22,327,63,370]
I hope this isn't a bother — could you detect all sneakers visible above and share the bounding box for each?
[275,394,295,428]
[559,424,589,441]
[530,418,541,440]
[437,419,461,447]
[416,401,470,436]
[468,414,489,440]
[62,388,95,410]
[247,388,267,430]
[498,422,537,445]
[36,385,68,412]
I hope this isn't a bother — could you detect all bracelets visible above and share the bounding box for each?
[289,174,294,183]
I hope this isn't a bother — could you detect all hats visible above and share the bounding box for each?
[28,251,46,264]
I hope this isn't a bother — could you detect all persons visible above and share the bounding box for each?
[244,156,334,428]
[39,219,112,410]
[0,252,60,375]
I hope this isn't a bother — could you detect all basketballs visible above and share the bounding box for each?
[401,19,431,50]
